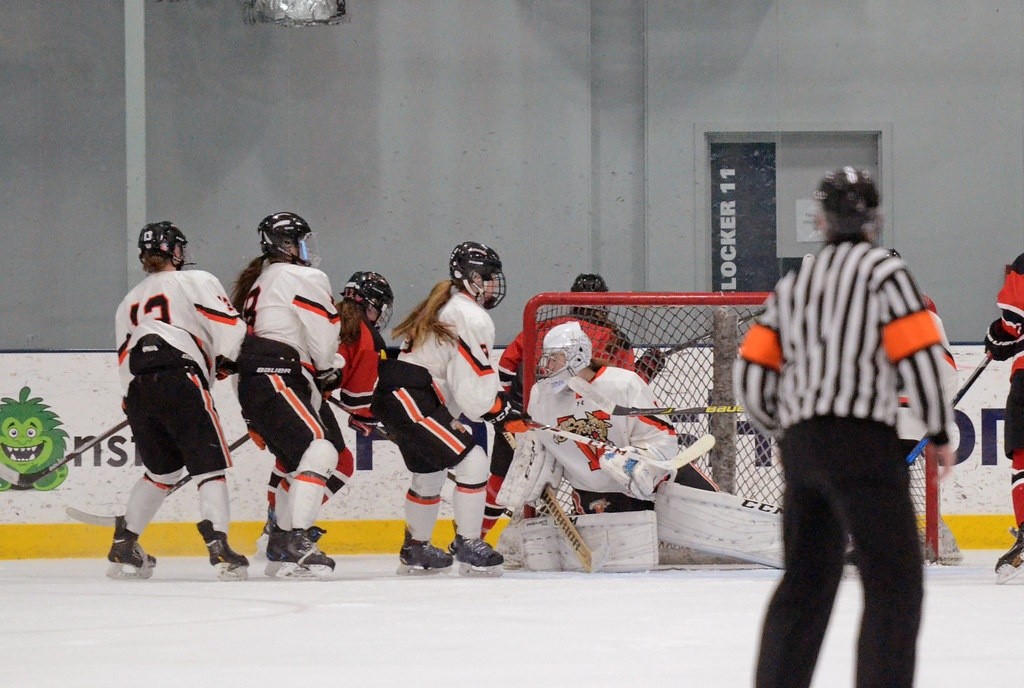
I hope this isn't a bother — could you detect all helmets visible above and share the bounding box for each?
[258,212,313,268]
[570,273,610,325]
[819,166,882,236]
[449,240,506,310]
[338,270,394,332]
[535,321,592,395]
[138,222,188,272]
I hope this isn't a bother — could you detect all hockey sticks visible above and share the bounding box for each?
[506,408,716,470]
[500,428,594,575]
[66,432,255,526]
[904,349,995,466]
[325,393,513,518]
[0,418,131,486]
[657,307,770,361]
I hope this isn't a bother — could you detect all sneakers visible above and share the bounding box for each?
[449,519,505,578]
[255,506,280,556]
[106,515,157,581]
[197,519,250,582]
[995,521,1024,584]
[265,525,284,576]
[396,525,454,576]
[276,526,336,580]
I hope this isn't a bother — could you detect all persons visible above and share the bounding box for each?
[481,274,665,540]
[734,167,960,688]
[496,321,719,572]
[241,271,393,563]
[379,242,531,577]
[107,222,249,581]
[984,252,1024,584]
[232,213,346,579]
[844,249,963,565]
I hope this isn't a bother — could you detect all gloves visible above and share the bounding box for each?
[215,354,237,379]
[984,317,1018,361]
[635,346,665,381]
[481,395,531,432]
